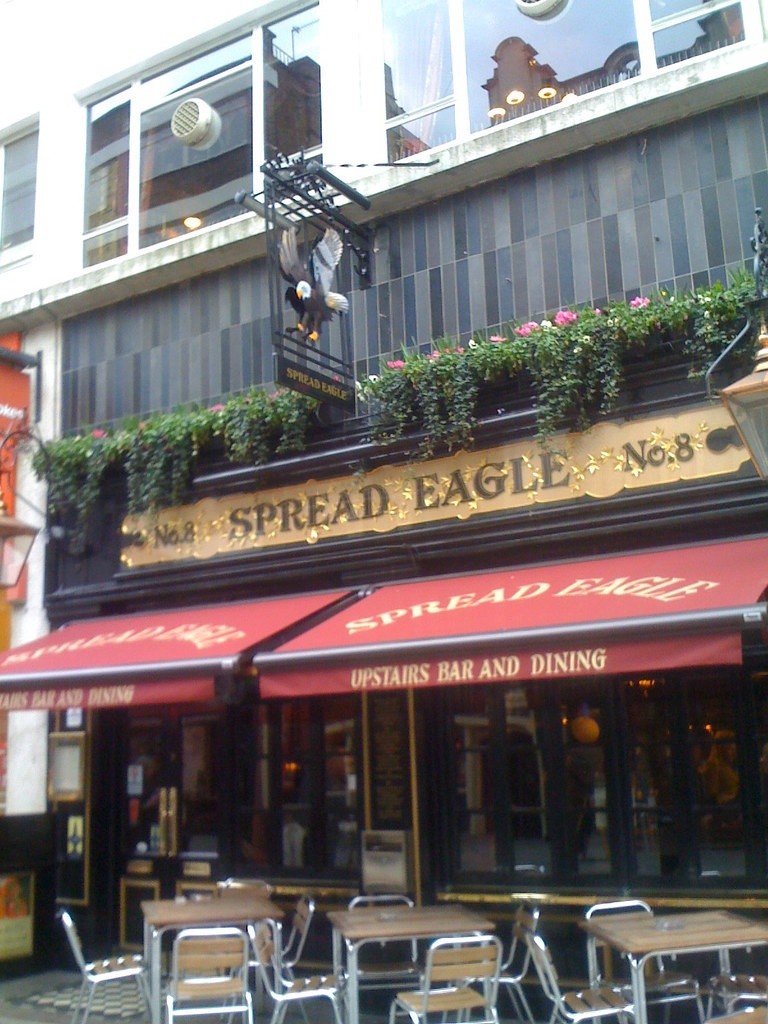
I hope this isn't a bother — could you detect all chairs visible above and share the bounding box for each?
[55,876,767,1024]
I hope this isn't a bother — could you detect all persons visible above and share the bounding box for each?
[702,730,739,840]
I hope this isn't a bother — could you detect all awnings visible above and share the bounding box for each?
[252,537,767,697]
[0,590,354,712]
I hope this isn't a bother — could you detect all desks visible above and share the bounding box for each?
[581,908,768,1024]
[326,905,498,1024]
[137,897,283,1024]
